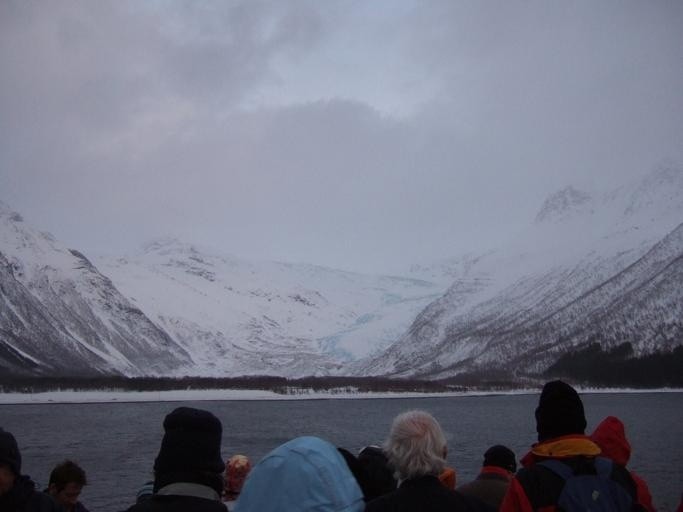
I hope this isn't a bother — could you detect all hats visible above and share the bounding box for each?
[535,379,587,438]
[153,406,226,477]
[483,444,516,472]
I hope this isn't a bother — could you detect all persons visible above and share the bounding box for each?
[436,465,456,491]
[136,480,155,505]
[221,452,253,511]
[364,409,470,512]
[358,445,398,500]
[587,415,658,511]
[458,445,519,511]
[230,434,367,511]
[42,459,92,511]
[1,426,54,511]
[501,380,649,511]
[124,407,231,509]
